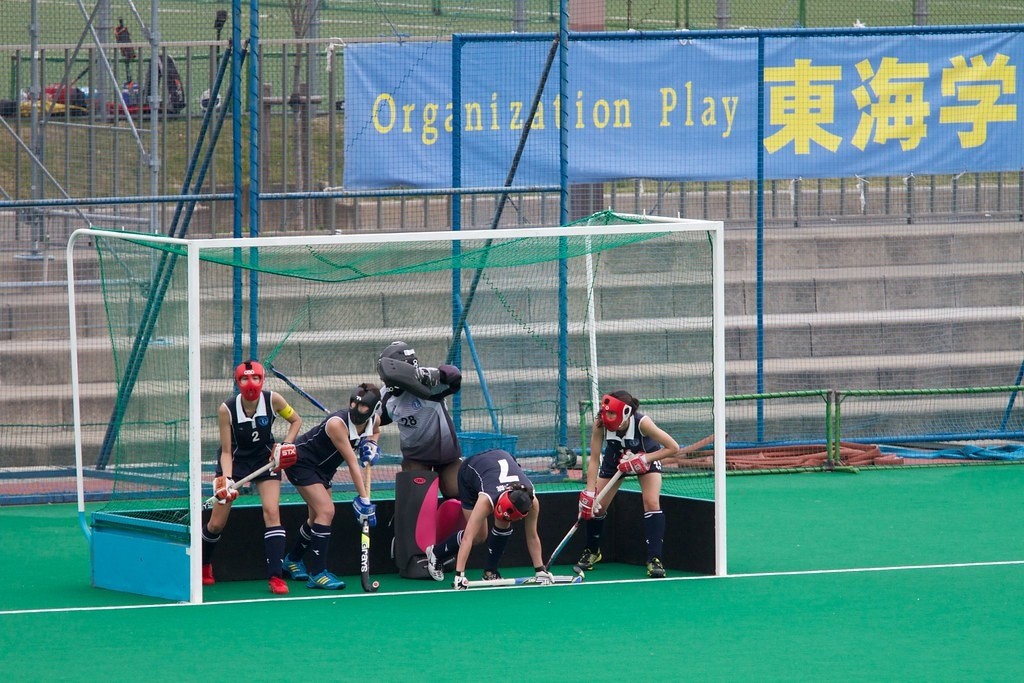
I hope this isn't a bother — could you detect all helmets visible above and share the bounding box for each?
[377,342,419,397]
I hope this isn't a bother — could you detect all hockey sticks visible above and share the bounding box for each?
[173,460,276,525]
[451,565,585,583]
[360,461,380,593]
[545,469,624,571]
[264,359,332,415]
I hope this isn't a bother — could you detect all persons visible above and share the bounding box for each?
[283,383,381,590]
[426,449,554,590]
[377,340,462,579]
[202,360,302,592]
[575,390,680,578]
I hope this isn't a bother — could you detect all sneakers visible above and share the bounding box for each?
[203,563,215,584]
[426,544,444,582]
[577,546,602,568]
[269,578,288,593]
[646,557,666,578]
[282,553,309,578]
[306,569,346,590]
[482,571,503,581]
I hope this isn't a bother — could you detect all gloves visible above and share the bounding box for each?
[578,490,602,520]
[268,443,297,476]
[359,440,380,468]
[617,450,650,475]
[212,477,238,505]
[353,495,372,529]
[416,367,441,387]
[535,567,555,586]
[454,571,468,590]
